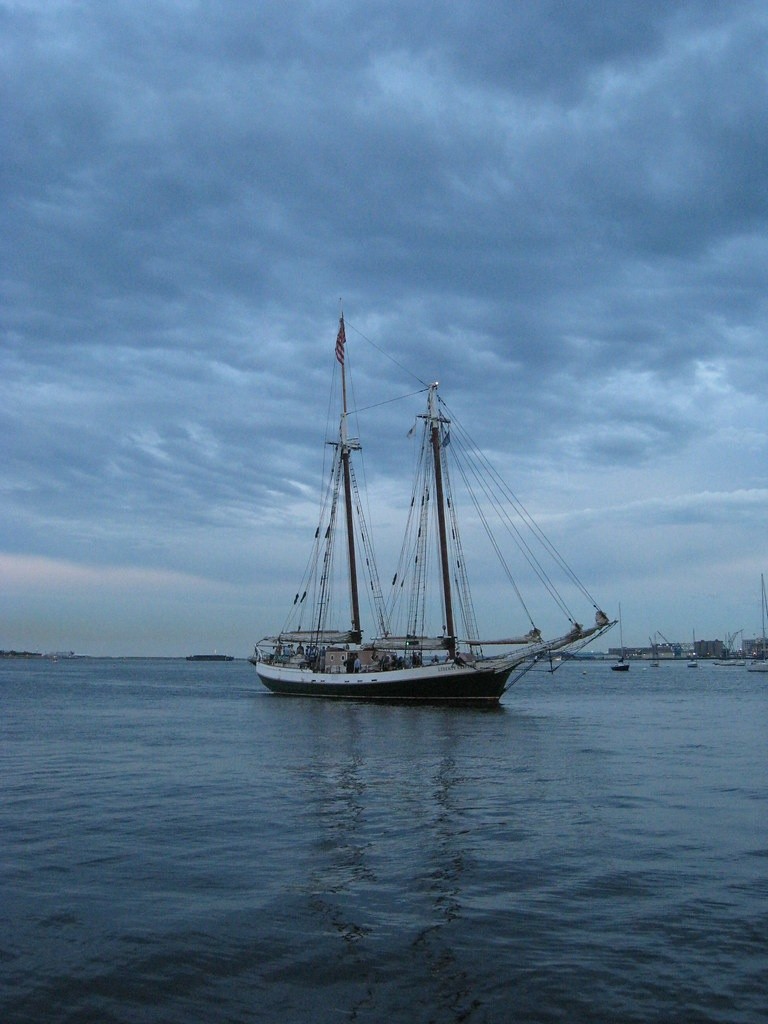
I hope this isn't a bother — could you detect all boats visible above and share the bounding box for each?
[610,657,629,671]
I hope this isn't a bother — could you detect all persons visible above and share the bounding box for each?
[271,641,439,674]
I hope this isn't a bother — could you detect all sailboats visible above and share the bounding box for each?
[245,312,622,710]
[644,575,767,672]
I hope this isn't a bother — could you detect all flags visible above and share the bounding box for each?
[406,424,417,439]
[335,311,347,365]
[440,423,451,448]
